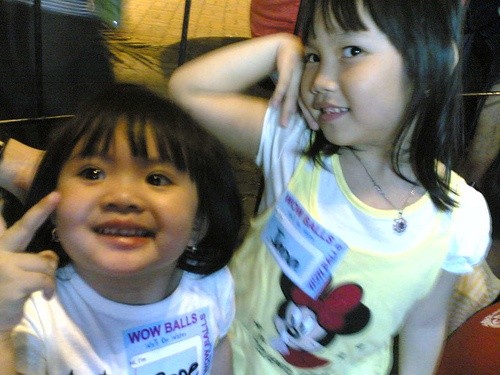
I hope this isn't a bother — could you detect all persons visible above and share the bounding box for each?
[1,0,500,206]
[170,0,490,375]
[1,83,248,375]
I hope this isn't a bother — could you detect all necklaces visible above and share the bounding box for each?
[347,148,419,232]
